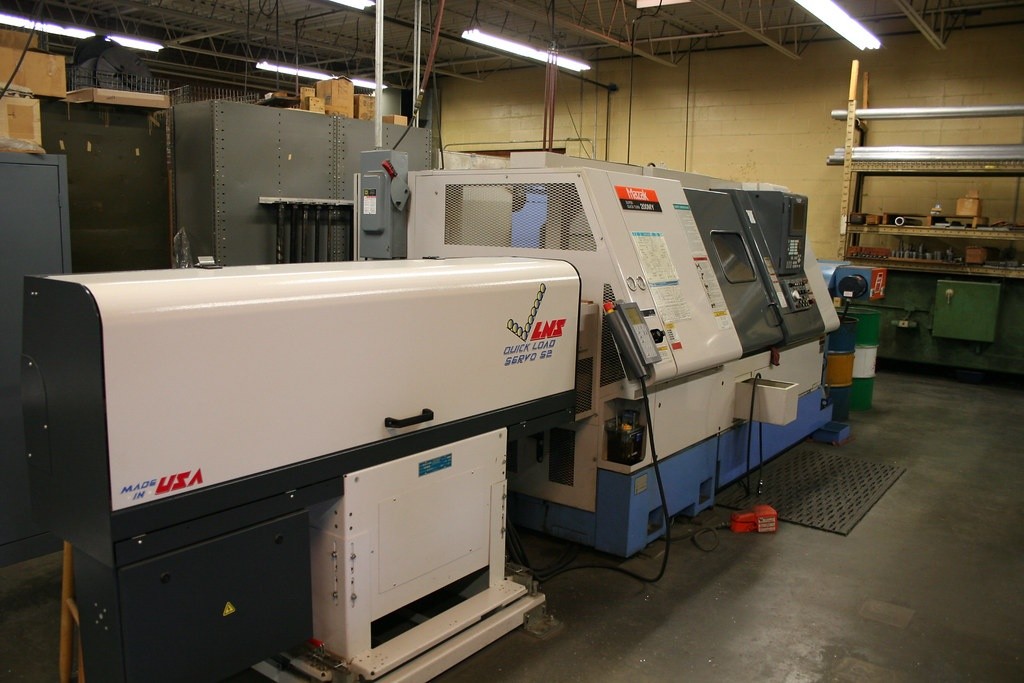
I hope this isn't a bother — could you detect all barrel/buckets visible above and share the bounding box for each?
[836,307,883,411]
[825,316,859,422]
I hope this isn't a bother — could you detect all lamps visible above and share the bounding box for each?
[255,58,388,90]
[460,26,594,73]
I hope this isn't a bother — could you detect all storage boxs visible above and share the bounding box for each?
[0,96,42,147]
[0,46,67,100]
[0,28,39,50]
[298,87,315,110]
[303,96,326,113]
[383,113,407,126]
[354,94,375,121]
[313,75,354,118]
[66,87,171,109]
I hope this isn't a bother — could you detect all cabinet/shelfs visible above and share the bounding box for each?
[837,61,1024,279]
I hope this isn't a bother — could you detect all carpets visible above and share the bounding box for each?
[715,447,904,538]
